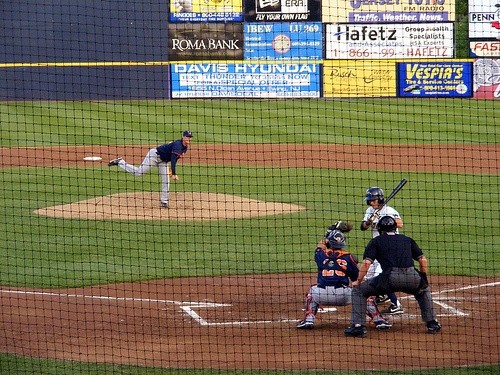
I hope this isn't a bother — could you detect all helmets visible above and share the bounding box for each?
[364,187,384,205]
[379,217,395,231]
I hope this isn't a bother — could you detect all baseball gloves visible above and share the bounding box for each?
[327,220,353,232]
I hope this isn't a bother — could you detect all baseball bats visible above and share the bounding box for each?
[367,179,408,226]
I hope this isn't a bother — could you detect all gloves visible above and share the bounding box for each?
[368,212,379,224]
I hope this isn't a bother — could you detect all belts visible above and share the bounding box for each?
[318,284,343,289]
[155,152,159,155]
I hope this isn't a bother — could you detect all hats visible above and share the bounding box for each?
[328,230,346,248]
[183,131,193,137]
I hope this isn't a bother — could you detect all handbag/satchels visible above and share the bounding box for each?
[370,269,392,293]
[417,269,428,289]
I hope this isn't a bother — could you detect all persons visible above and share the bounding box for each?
[107,131,194,208]
[297,221,392,329]
[343,215,441,336]
[361,187,404,313]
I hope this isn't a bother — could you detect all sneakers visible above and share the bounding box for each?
[344,325,366,338]
[427,322,442,333]
[374,319,393,329]
[161,203,167,208]
[108,156,122,166]
[380,301,404,314]
[296,319,313,328]
